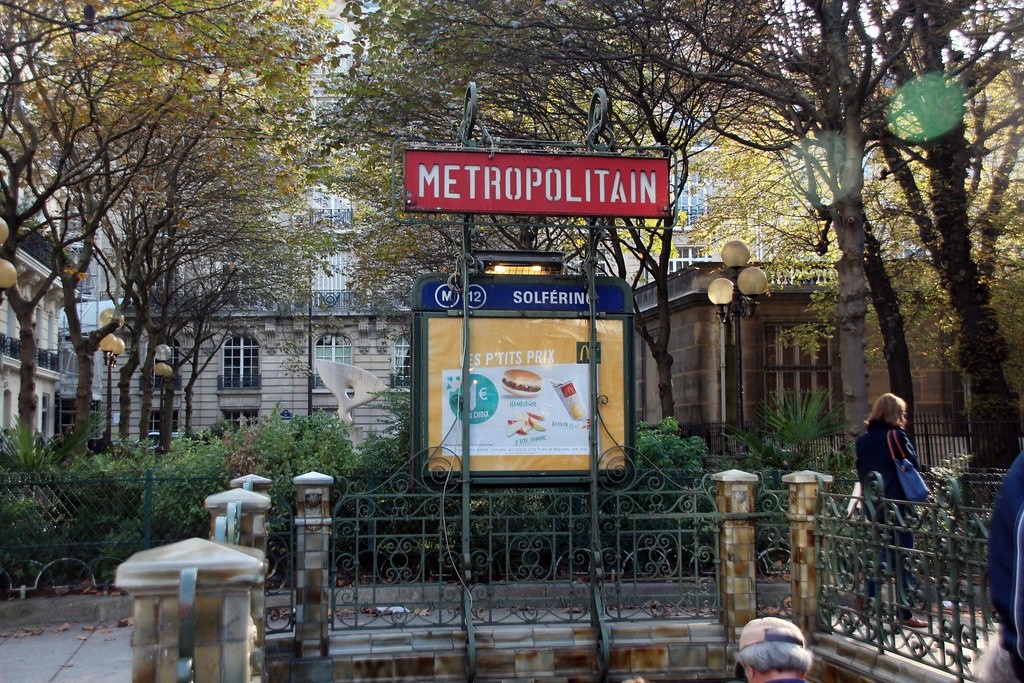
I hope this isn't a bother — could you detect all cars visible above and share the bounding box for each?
[135,432,160,452]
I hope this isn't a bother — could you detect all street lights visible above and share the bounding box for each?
[100,309,125,451]
[707,239,768,452]
[155,344,172,452]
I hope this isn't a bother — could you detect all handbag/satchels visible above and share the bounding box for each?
[886,429,932,502]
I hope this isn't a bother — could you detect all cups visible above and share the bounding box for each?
[553,379,586,421]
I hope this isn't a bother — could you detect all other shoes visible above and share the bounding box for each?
[903,616,929,627]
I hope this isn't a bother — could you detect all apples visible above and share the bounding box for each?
[506,411,546,436]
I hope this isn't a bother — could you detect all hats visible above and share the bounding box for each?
[734,616,806,681]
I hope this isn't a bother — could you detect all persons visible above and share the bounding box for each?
[734,617,813,683]
[855,392,930,627]
[987,451,1024,683]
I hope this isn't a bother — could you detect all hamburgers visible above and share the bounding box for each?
[501,369,542,397]
